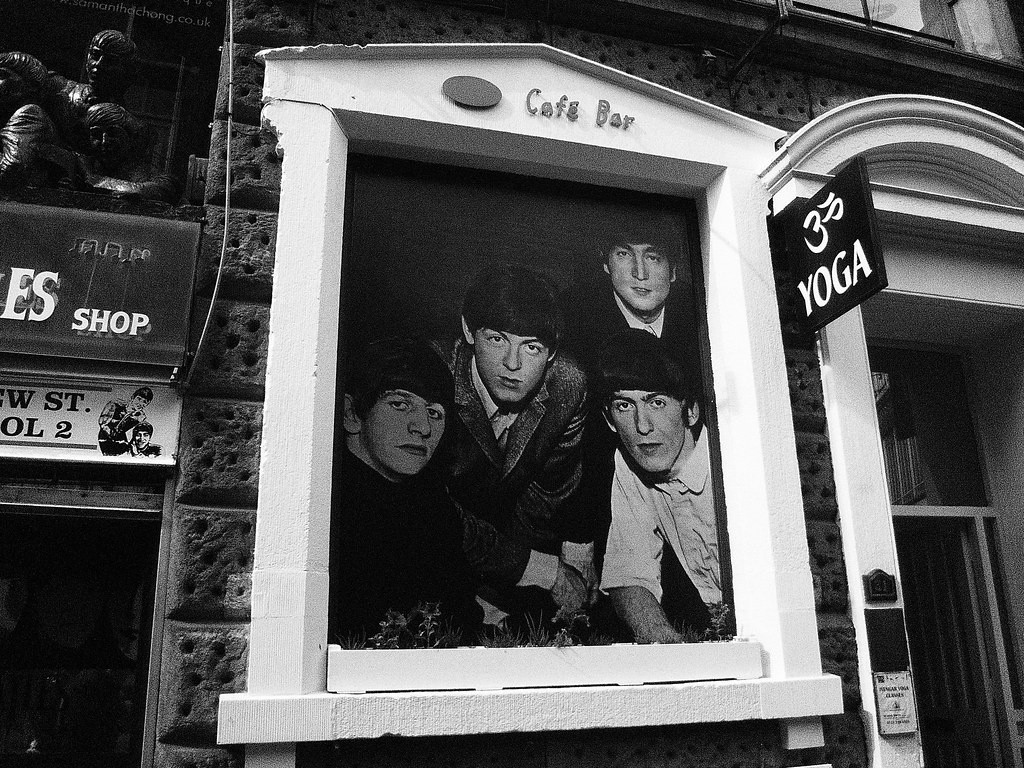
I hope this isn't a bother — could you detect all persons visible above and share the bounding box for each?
[584,327,729,644]
[430,266,598,647]
[0,51,57,187]
[552,205,705,461]
[86,103,180,207]
[48,30,140,152]
[326,336,485,649]
